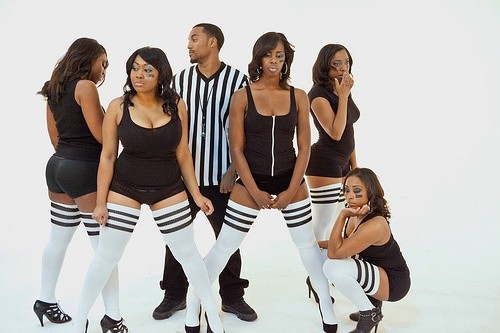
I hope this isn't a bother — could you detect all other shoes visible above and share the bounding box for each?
[222,300,257,321]
[152,296,186,320]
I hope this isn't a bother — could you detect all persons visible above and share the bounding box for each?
[151,23,259,321]
[306,45,360,304]
[33,38,129,333]
[184,32,338,333]
[73,46,227,333]
[316,168,412,333]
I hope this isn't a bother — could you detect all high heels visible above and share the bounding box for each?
[185,305,202,333]
[319,303,338,333]
[34,300,72,327]
[348,306,383,333]
[349,300,382,322]
[101,314,128,333]
[85,320,89,333]
[305,275,334,304]
[205,312,226,333]
